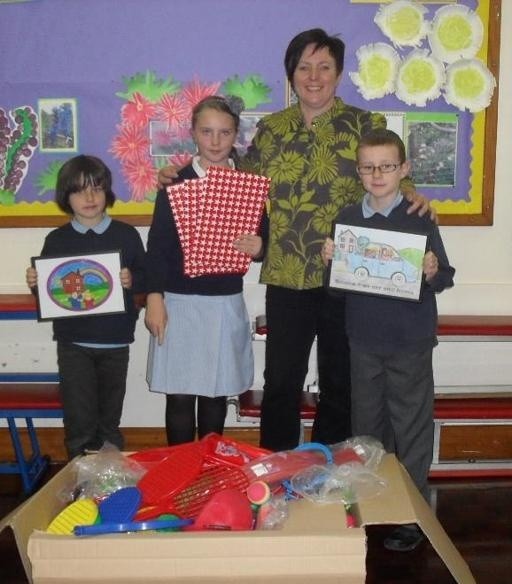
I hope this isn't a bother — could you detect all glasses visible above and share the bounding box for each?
[357,164,402,174]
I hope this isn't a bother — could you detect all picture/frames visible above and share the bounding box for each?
[0,0,503,229]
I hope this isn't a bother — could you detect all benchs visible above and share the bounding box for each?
[0,383,66,495]
[237,387,512,477]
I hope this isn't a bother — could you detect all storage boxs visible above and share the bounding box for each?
[0,451,479,584]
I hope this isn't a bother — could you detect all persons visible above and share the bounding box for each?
[27,155,146,461]
[319,129,455,552]
[157,29,439,451]
[145,94,270,447]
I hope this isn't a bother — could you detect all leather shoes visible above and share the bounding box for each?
[382,526,424,550]
